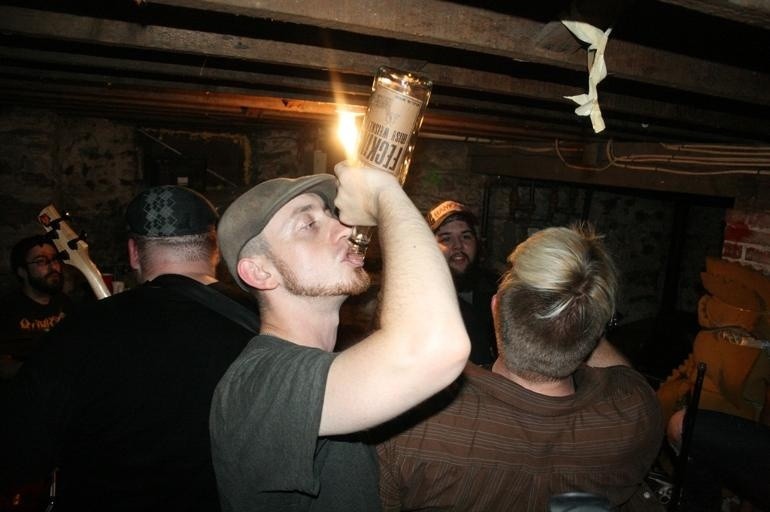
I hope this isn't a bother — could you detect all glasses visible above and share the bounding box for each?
[28,254,61,264]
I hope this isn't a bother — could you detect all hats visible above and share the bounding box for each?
[426,200,478,231]
[126,185,219,236]
[217,174,336,293]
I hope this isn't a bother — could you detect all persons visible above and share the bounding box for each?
[664,408,770,511]
[375,222,667,511]
[424,197,502,370]
[209,159,474,512]
[0,183,262,511]
[2,235,79,363]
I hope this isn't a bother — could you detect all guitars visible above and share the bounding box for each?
[38,205,110,300]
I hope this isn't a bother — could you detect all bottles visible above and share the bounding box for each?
[346,64,433,262]
